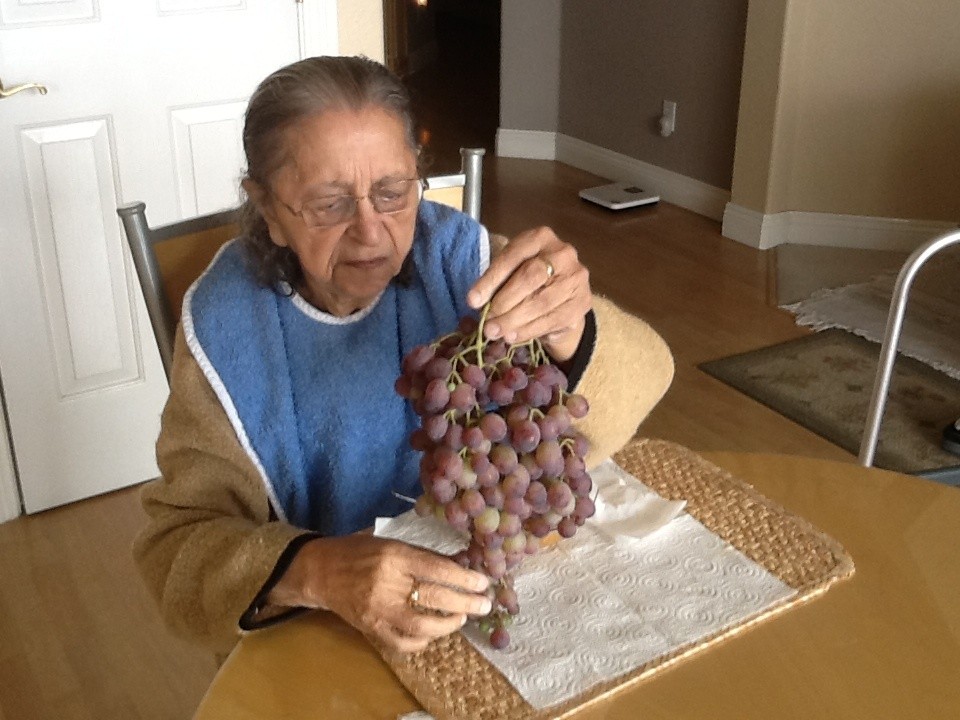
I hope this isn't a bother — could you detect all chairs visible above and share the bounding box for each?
[116,146,488,388]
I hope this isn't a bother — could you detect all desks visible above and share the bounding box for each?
[194,451,959,719]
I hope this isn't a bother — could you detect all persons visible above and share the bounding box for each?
[130,57,677,655]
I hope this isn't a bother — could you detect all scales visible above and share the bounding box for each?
[579,181,660,213]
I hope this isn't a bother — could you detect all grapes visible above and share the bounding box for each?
[395,317,595,647]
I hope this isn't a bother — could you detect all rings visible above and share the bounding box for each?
[404,580,421,609]
[537,254,555,287]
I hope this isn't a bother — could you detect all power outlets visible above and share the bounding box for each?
[662,100,676,131]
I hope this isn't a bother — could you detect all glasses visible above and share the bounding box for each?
[261,157,430,229]
